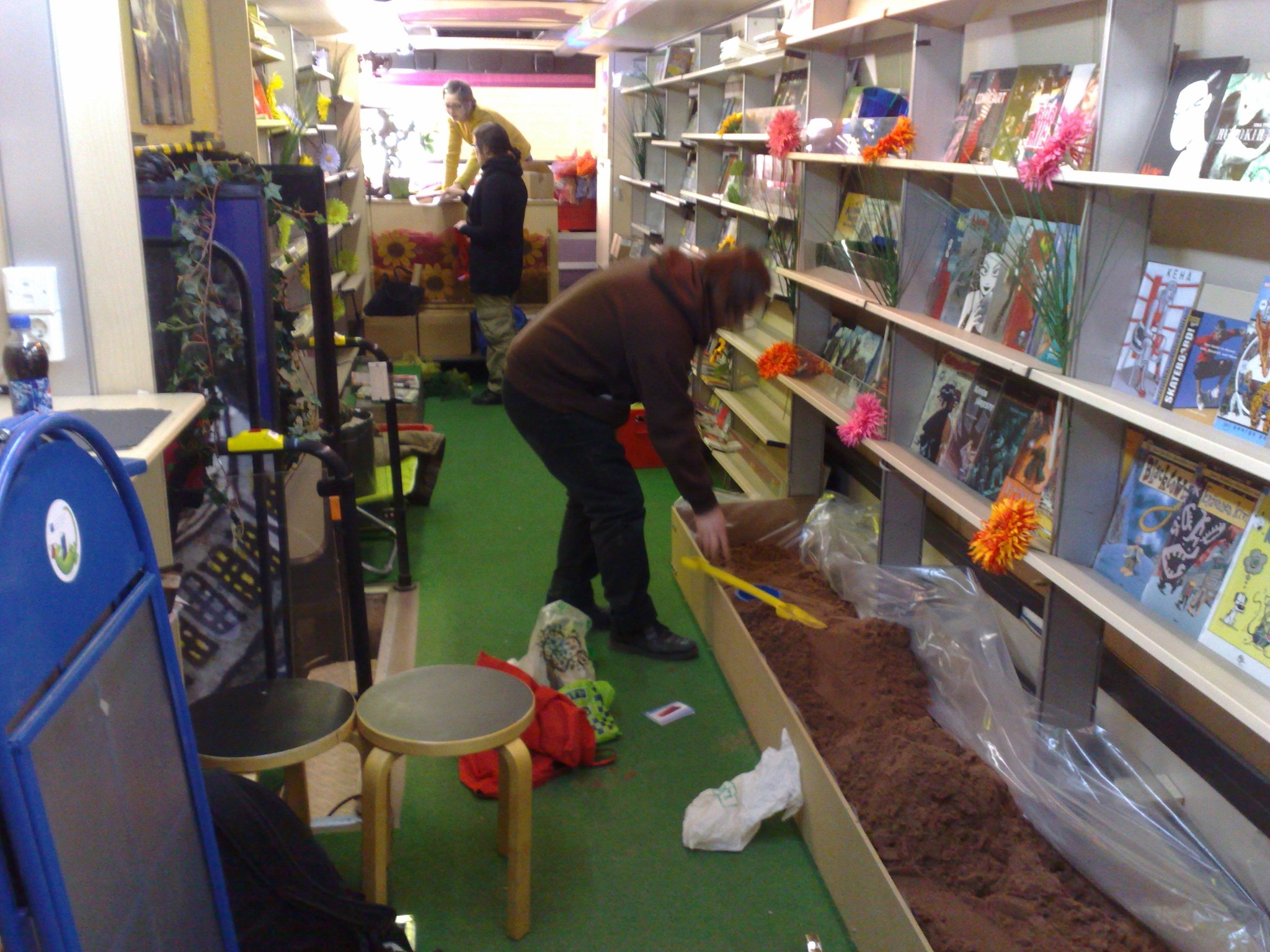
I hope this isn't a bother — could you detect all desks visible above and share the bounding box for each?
[0,392,207,482]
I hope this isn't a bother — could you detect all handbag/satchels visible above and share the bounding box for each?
[460,600,617,797]
[363,265,426,316]
[681,729,803,852]
[334,410,377,497]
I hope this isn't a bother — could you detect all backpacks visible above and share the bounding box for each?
[198,766,396,952]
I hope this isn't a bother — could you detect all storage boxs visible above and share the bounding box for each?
[669,497,1263,952]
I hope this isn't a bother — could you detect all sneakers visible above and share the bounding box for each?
[580,606,615,632]
[608,620,700,661]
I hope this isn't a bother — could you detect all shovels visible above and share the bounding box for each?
[736,584,778,606]
[680,553,828,633]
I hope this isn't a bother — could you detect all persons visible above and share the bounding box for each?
[441,123,527,405]
[443,79,533,191]
[502,246,770,660]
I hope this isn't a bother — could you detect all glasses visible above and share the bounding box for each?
[446,101,466,109]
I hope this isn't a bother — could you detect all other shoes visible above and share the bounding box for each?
[473,388,504,406]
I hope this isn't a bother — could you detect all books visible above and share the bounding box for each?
[614,31,1270,687]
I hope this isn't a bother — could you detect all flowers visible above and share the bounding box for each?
[861,113,920,164]
[963,489,1036,570]
[763,108,808,161]
[713,107,746,139]
[835,388,889,445]
[1016,102,1098,193]
[753,337,831,382]
[263,69,359,292]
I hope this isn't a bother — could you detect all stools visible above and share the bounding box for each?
[186,679,397,870]
[354,664,537,940]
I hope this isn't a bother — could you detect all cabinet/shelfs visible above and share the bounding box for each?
[616,0,1270,783]
[209,0,377,403]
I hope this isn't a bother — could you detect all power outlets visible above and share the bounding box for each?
[3,265,61,316]
[22,313,66,363]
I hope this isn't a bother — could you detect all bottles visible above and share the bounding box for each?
[2,315,53,415]
